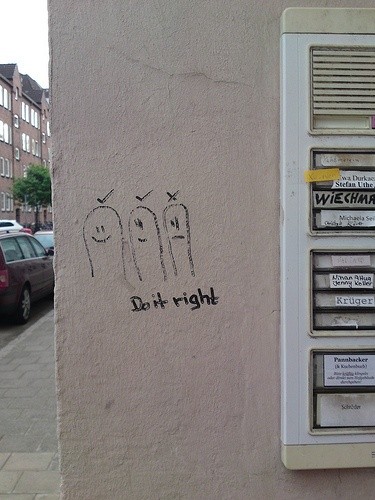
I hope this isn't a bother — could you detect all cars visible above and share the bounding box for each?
[0,228,54,323]
[0,219,24,233]
[31,231,54,266]
